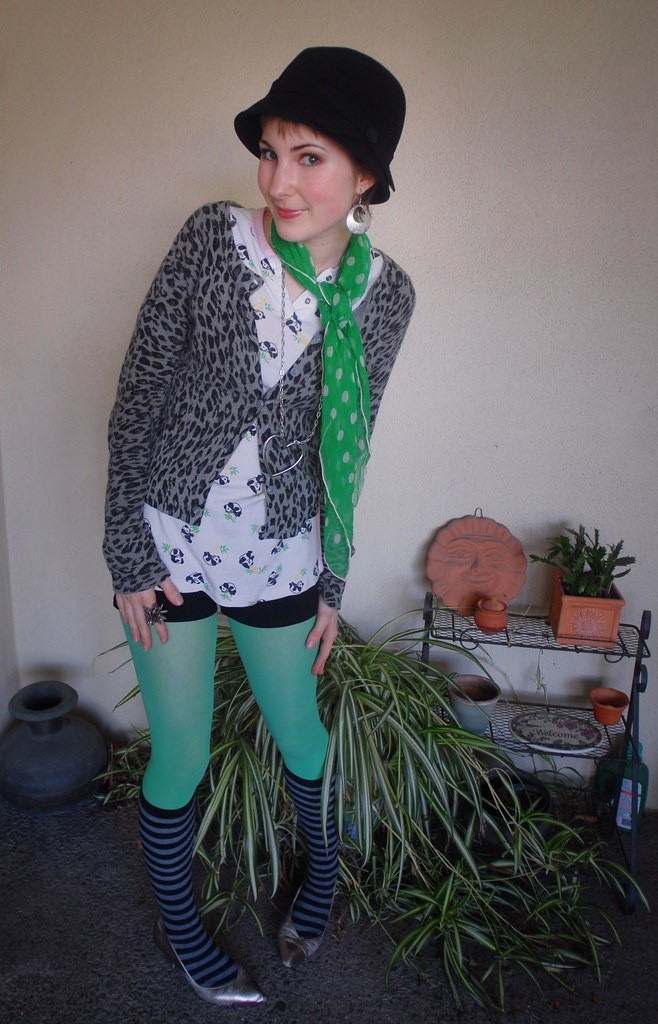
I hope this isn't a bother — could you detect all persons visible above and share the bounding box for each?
[101,47,417,1005]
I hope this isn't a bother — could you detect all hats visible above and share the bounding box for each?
[234,47,406,204]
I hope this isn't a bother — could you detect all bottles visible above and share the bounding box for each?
[595,741,649,834]
[0,680,108,811]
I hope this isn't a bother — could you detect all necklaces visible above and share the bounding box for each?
[263,266,326,476]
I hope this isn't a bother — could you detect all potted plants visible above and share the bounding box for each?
[94,608,651,1024]
[529,524,635,648]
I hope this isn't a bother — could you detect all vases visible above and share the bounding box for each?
[446,674,501,736]
[590,686,630,726]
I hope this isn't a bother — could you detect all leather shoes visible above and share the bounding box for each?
[277,872,336,967]
[154,915,267,1004]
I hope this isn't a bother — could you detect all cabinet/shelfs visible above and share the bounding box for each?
[419,592,652,912]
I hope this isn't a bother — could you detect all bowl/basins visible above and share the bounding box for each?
[448,674,501,735]
[588,687,629,725]
[475,764,550,828]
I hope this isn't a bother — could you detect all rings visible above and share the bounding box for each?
[143,602,168,625]
